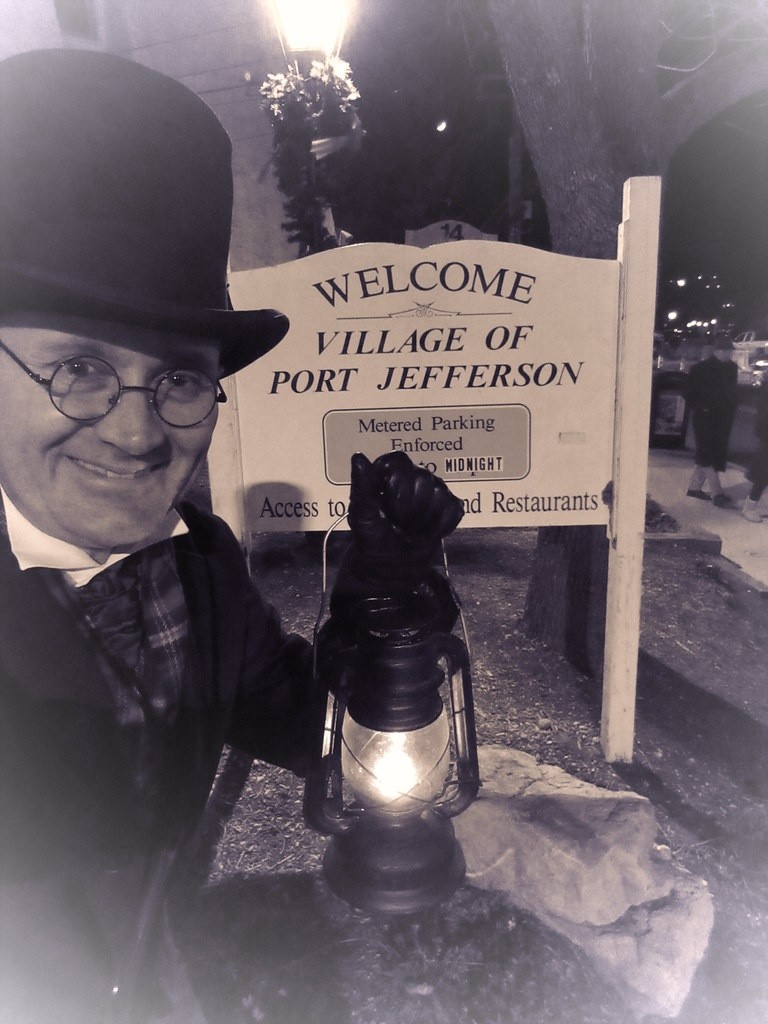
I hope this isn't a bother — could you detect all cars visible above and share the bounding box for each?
[731,329,768,351]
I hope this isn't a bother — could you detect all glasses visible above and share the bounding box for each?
[0,341,227,428]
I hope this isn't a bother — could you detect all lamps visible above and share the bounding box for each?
[299,496,486,920]
[261,1,360,87]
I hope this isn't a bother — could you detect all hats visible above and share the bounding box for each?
[2,49,292,380]
[709,336,736,351]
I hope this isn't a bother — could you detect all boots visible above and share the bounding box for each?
[756,507,768,518]
[742,496,763,523]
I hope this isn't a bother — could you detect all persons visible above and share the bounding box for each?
[0,48,469,1024]
[686,336,740,506]
[740,356,768,523]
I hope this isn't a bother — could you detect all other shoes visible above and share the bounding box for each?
[687,490,712,500]
[713,494,730,506]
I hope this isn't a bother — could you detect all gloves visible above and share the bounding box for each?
[349,449,463,581]
